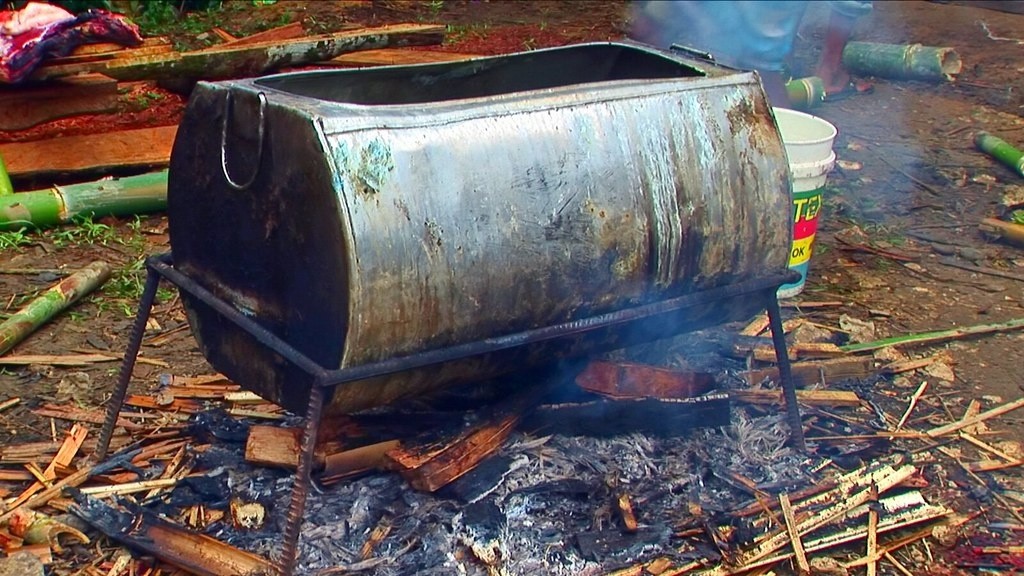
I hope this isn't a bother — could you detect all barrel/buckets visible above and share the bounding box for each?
[769,107,837,298]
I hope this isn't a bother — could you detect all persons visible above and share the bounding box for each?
[629,0,876,110]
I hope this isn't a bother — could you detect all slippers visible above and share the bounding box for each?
[823,74,873,103]
